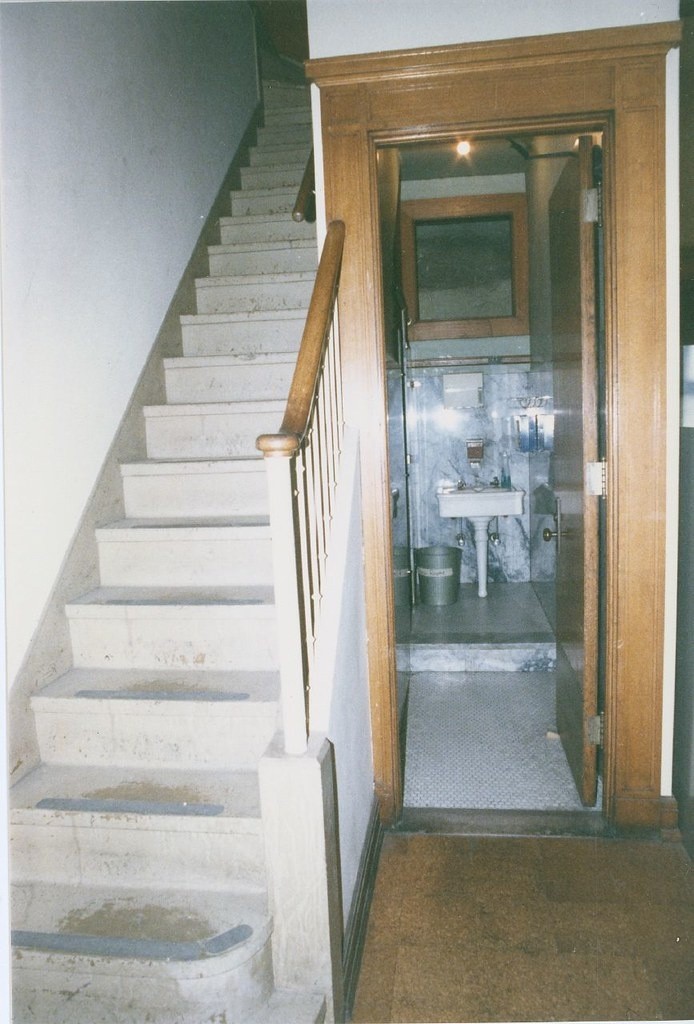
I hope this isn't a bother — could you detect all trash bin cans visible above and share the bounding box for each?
[414,545,463,607]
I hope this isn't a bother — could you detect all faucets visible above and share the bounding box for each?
[490,476,500,487]
[457,480,466,490]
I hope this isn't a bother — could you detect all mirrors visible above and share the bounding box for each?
[442,372,485,410]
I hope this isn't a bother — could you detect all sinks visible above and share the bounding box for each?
[435,486,525,518]
